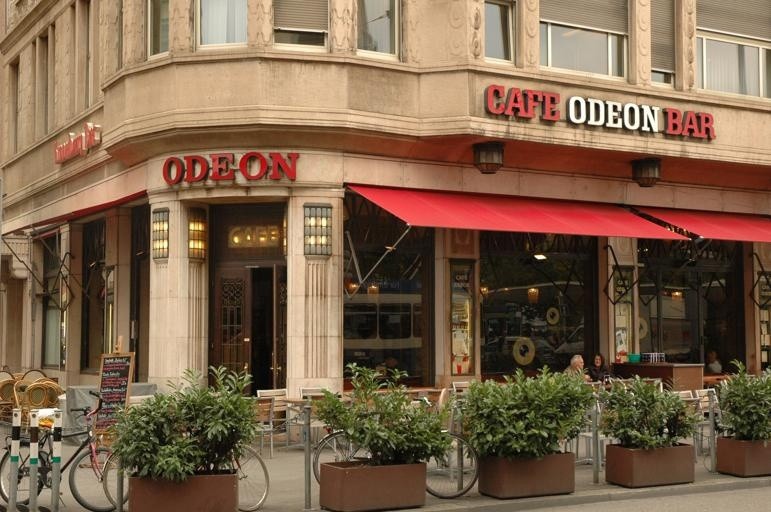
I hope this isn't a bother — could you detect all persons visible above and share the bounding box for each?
[588,351,611,386]
[385,354,415,376]
[563,354,595,382]
[706,348,724,373]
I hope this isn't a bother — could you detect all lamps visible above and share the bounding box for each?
[632,160,661,187]
[473,146,504,174]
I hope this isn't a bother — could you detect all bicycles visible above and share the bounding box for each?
[102,445,269,512]
[0,390,129,512]
[313,398,480,499]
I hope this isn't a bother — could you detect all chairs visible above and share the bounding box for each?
[713,359,771,477]
[251,387,337,459]
[566,380,721,462]
[0,370,64,435]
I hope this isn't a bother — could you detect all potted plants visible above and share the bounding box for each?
[458,365,598,499]
[598,373,706,488]
[305,362,458,512]
[105,364,263,512]
